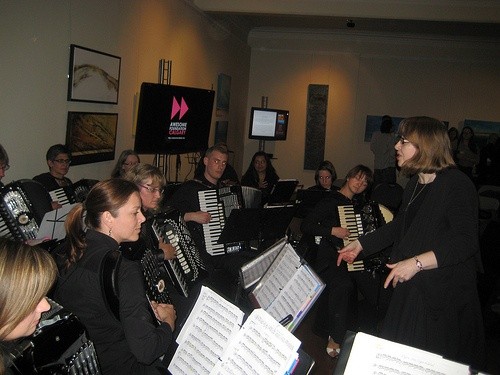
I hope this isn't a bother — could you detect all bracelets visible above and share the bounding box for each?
[414,256,424,271]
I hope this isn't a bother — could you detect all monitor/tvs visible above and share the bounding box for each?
[134,82,216,155]
[248,106,289,141]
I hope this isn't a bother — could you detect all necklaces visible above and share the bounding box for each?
[405,173,435,212]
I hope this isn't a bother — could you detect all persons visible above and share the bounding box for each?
[336,117,485,371]
[0,146,49,246]
[302,160,337,256]
[449,126,481,180]
[241,152,280,196]
[124,162,177,263]
[32,144,72,210]
[54,179,177,375]
[371,115,398,184]
[165,145,239,276]
[300,165,388,358]
[111,150,140,179]
[0,237,59,375]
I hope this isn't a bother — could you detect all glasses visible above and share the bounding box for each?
[0,166,8,171]
[137,184,164,193]
[125,162,137,165]
[399,136,408,144]
[55,159,71,164]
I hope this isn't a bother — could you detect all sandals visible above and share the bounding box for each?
[326,336,340,357]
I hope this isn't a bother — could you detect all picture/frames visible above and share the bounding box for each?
[67,44,121,103]
[65,111,118,165]
